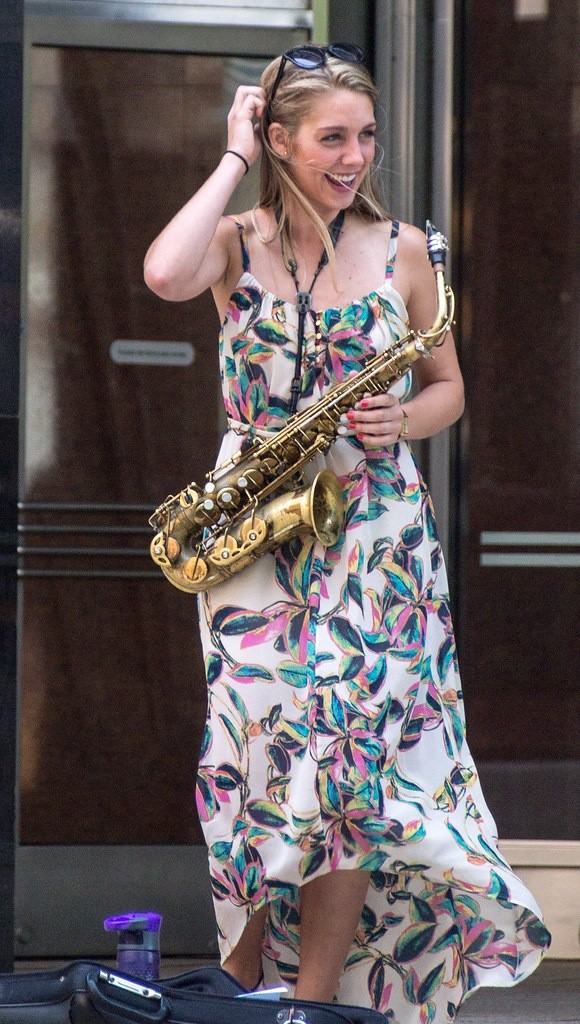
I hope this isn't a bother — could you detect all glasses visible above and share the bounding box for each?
[266,42,369,131]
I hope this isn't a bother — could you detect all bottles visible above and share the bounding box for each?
[104,912,163,981]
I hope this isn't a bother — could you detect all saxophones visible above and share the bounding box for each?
[147,220,459,594]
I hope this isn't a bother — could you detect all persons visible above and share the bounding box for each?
[143,41,552,1024]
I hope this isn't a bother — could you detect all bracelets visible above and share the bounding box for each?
[224,151,249,175]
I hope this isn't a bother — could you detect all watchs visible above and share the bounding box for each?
[397,409,409,442]
